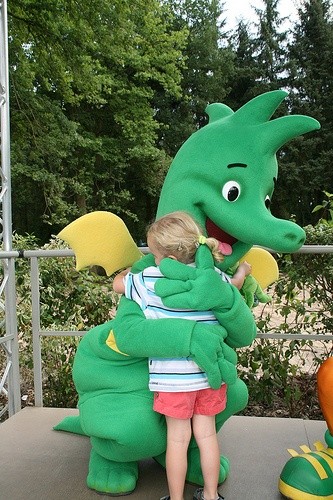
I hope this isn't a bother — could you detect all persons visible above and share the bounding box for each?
[113,211,252,499]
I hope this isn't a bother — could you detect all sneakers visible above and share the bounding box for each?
[160,495,171,500]
[194,488,225,500]
